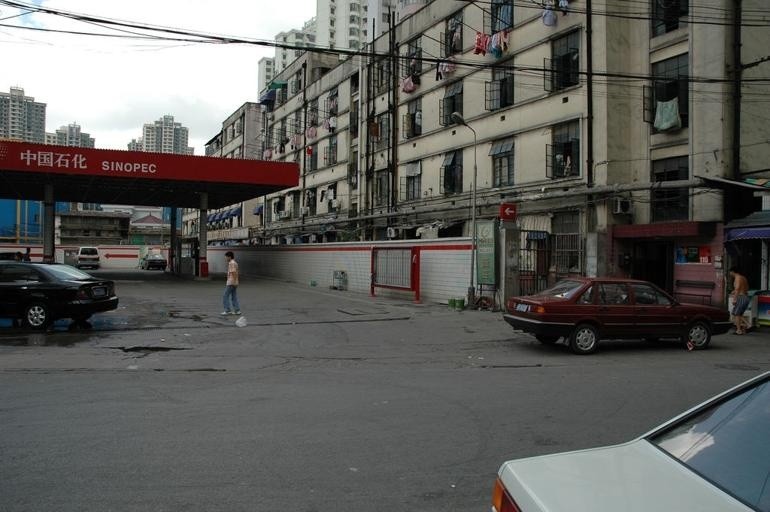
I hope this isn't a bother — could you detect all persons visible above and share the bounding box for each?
[14,251,24,263]
[219,250,242,315]
[24,247,32,262]
[727,266,753,335]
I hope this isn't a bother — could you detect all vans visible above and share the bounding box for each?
[72,246,101,269]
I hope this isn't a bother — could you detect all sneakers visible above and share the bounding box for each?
[220,310,242,316]
[732,328,746,335]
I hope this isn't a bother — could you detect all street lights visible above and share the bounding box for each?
[451,110,477,309]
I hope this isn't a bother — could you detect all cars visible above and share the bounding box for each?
[140,254,168,270]
[489,368,769,512]
[502,276,733,355]
[0,261,120,332]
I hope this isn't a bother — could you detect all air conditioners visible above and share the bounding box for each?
[279,199,340,219]
[387,227,398,238]
